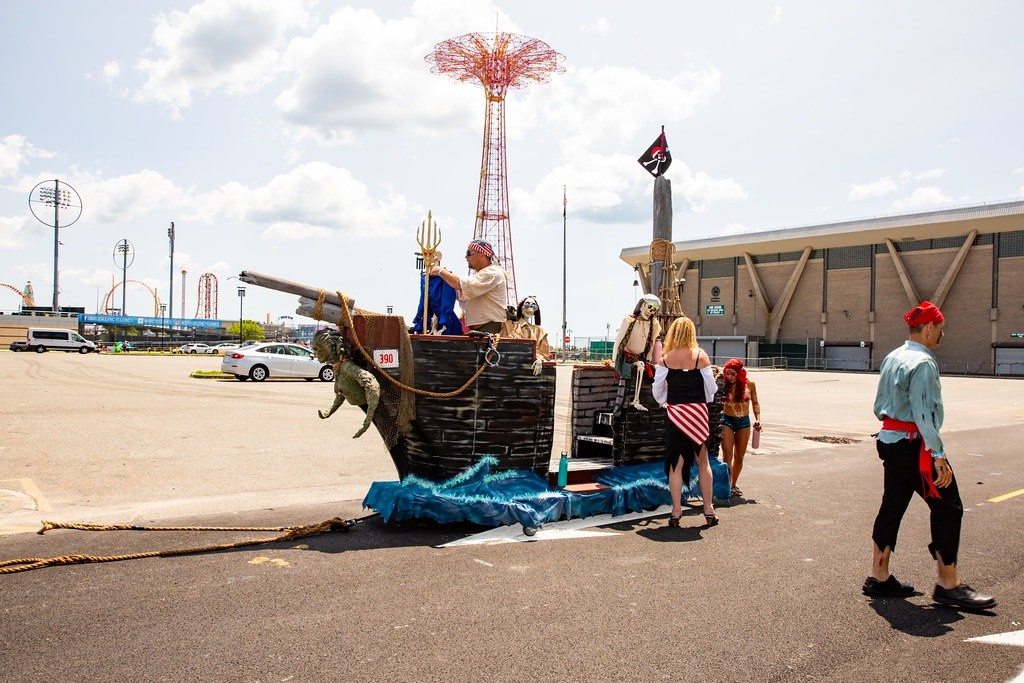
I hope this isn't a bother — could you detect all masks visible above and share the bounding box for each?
[929,329,944,344]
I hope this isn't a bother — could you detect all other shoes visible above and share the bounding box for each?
[731,486,742,496]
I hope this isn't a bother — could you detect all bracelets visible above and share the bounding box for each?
[930,449,945,457]
[438,269,444,276]
[934,453,947,459]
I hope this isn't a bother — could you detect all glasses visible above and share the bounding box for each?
[466,251,477,256]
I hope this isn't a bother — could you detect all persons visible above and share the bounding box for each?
[653,316,719,526]
[412,250,463,341]
[313,328,408,482]
[719,358,763,496]
[497,296,550,376]
[122,338,130,352]
[430,240,508,336]
[863,300,998,609]
[710,365,719,378]
[607,294,663,412]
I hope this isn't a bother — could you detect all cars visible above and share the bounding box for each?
[172,340,261,354]
[221,344,334,382]
[9,340,36,352]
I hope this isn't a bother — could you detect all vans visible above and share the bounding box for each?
[26,327,95,354]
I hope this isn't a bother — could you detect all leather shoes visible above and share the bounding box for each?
[863,574,915,596]
[933,582,997,609]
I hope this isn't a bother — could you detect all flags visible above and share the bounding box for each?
[638,130,672,178]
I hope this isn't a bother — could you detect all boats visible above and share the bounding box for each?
[240,125,725,485]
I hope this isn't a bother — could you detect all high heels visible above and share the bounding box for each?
[670,516,682,527]
[705,512,720,526]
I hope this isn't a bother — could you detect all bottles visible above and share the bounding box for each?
[752,422,761,449]
[652,335,662,363]
[558,452,569,487]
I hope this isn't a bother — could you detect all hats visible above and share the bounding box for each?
[904,300,944,330]
[469,240,494,257]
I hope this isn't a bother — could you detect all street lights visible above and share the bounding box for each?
[113,238,135,316]
[28,179,83,312]
[160,303,167,350]
[167,221,176,338]
[236,286,247,344]
[566,329,572,351]
